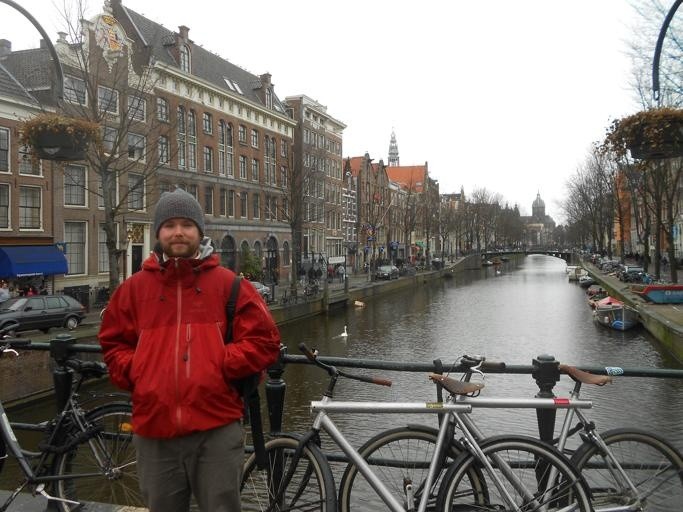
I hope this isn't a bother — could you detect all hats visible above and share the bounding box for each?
[154,188,205,239]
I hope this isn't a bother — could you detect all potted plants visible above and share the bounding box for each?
[12,110,106,165]
[592,106,683,170]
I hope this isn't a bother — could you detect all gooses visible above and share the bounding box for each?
[341,326,348,336]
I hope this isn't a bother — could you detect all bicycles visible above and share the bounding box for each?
[336,352,683,512]
[0,323,144,512]
[280,288,295,304]
[240,342,594,512]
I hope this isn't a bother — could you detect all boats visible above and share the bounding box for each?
[481,256,512,266]
[566,266,641,331]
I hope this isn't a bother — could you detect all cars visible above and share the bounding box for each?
[0,294,87,337]
[371,264,399,281]
[251,281,271,303]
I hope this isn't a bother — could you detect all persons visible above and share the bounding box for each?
[298,262,345,287]
[96,186,283,512]
[0,282,10,303]
[20,283,47,298]
